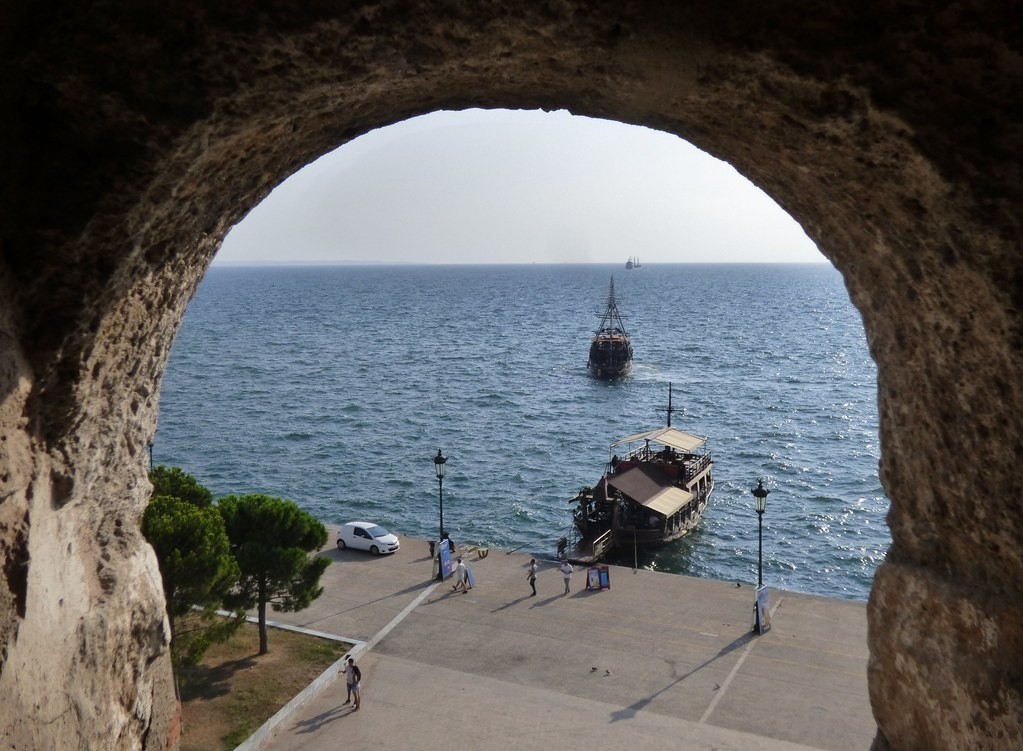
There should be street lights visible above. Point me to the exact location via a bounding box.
[750,479,772,634]
[431,449,450,580]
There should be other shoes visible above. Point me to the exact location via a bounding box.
[564,593,566,595]
[567,591,570,593]
[463,590,467,593]
[452,585,457,590]
[531,592,536,596]
[354,706,359,710]
[344,700,351,705]
[352,704,356,707]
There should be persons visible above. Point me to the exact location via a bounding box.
[452,559,467,593]
[560,560,573,594]
[527,559,537,596]
[612,446,684,529]
[338,659,362,711]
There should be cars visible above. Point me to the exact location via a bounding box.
[335,522,400,556]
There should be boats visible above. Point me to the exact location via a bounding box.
[625,256,642,270]
[587,273,634,380]
[568,382,715,548]
